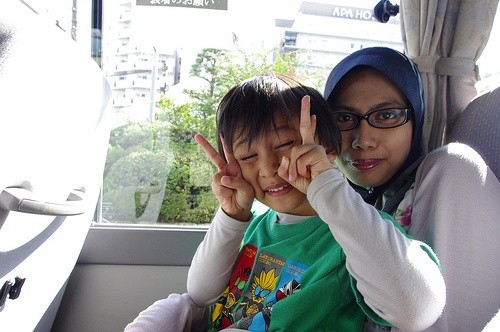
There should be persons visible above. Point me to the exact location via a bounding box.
[186,73,447,332]
[121,47,500,332]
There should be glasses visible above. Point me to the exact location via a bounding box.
[329,106,413,132]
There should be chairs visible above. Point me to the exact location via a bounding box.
[0,0,114,332]
[393,86,500,331]
